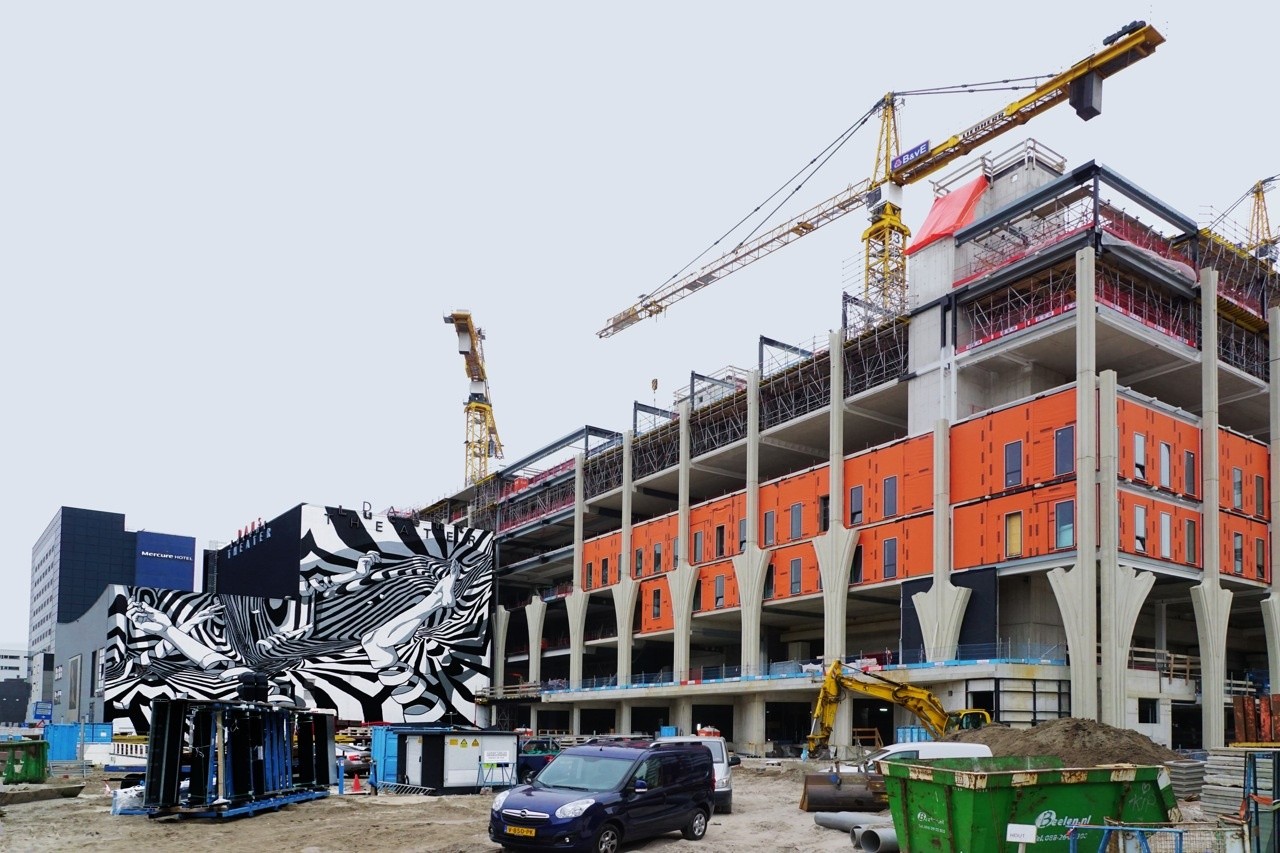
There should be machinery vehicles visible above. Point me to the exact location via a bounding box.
[805,660,993,764]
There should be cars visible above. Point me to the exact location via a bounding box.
[292,742,371,778]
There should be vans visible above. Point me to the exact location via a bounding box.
[658,736,742,813]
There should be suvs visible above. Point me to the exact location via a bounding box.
[487,740,717,853]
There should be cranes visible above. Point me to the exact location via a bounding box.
[596,18,1167,323]
[441,310,504,489]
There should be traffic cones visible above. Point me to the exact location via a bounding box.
[351,774,364,792]
